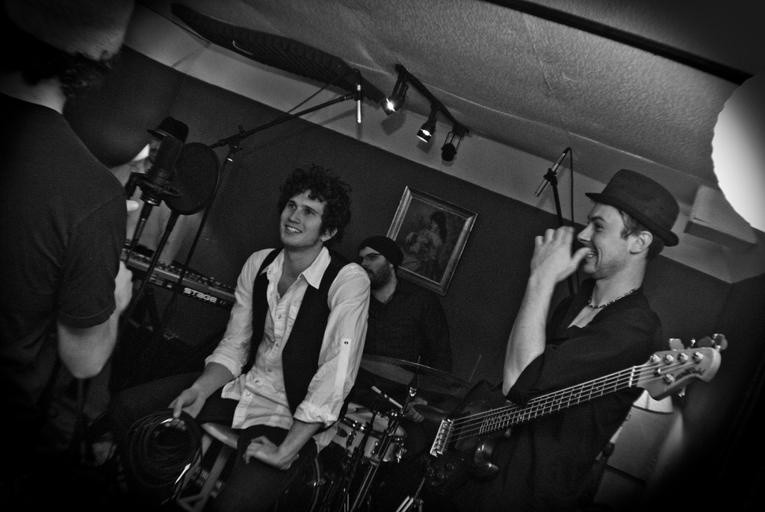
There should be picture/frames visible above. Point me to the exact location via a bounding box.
[385,184,479,295]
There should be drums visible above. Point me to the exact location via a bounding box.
[282,452,341,510]
[334,402,407,464]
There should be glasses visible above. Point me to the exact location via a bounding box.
[358,252,385,262]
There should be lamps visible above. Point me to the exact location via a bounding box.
[384,63,470,162]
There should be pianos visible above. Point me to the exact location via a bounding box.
[121,246,235,308]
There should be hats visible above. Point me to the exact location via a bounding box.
[357,235,402,264]
[4,0,136,65]
[147,116,189,146]
[584,168,680,247]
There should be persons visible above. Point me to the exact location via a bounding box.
[1,1,135,511]
[320,235,455,512]
[117,164,373,510]
[399,209,449,278]
[457,170,679,510]
[108,117,192,315]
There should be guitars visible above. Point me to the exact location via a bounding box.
[420,332,727,494]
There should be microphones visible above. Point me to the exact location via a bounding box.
[534,146,570,198]
[136,135,184,204]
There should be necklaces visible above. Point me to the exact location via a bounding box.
[585,288,636,310]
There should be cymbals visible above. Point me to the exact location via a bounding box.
[363,353,470,395]
[413,404,448,422]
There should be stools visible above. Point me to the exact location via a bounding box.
[160,421,240,512]
